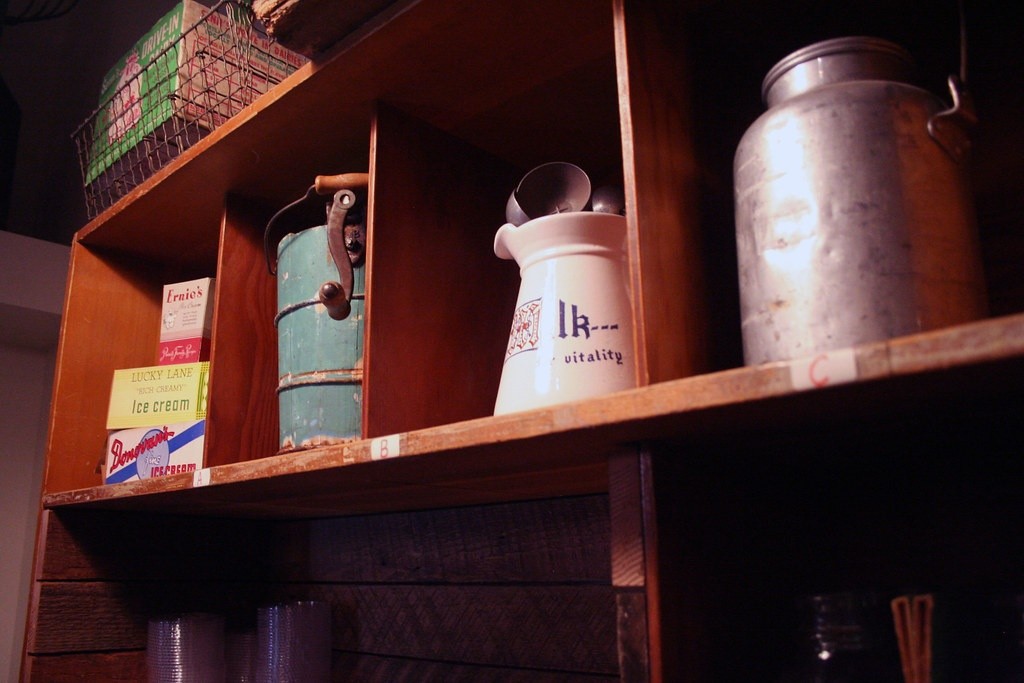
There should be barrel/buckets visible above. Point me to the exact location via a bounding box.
[729,36,987,369]
[263,173,372,452]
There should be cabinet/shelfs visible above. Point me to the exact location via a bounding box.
[20,0,1024,683]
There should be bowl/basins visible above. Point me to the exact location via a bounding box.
[147,597,338,683]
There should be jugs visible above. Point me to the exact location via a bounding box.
[488,210,640,418]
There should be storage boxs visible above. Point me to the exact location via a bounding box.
[65,2,983,485]
